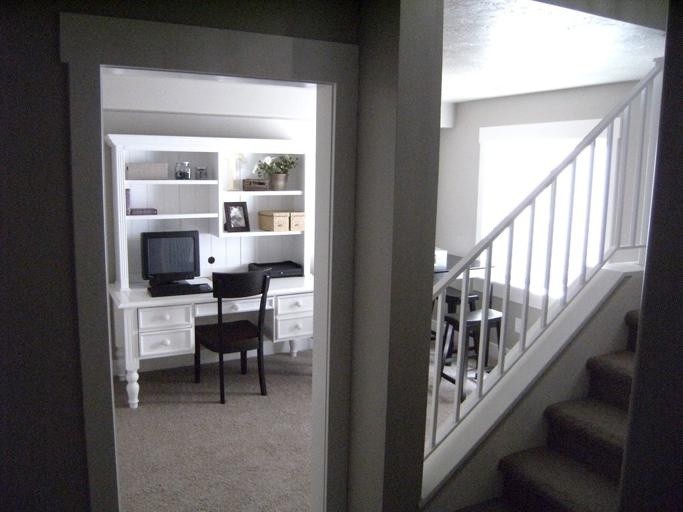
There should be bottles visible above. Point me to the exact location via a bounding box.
[174,161,209,180]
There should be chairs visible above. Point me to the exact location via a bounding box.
[194,267,273,404]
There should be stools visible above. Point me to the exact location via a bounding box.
[445,286,479,366]
[441,308,503,403]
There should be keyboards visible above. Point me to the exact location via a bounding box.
[148,284,212,297]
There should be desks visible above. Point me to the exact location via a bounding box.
[434,253,495,273]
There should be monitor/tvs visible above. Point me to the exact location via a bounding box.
[141,231,200,286]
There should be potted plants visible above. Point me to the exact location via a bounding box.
[258,155,299,189]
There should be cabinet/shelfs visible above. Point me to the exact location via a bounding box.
[105,133,315,410]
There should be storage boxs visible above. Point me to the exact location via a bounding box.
[289,211,305,231]
[258,210,289,232]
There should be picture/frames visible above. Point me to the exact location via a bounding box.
[224,202,251,233]
